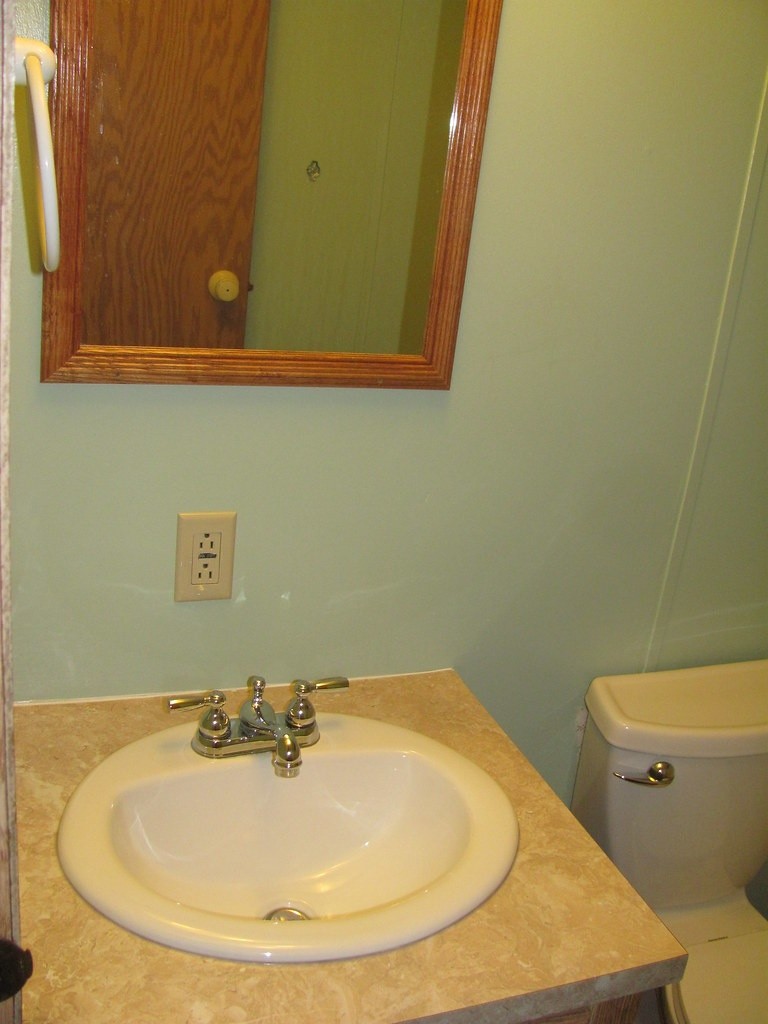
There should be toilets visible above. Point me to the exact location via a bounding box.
[569,659,768,1023]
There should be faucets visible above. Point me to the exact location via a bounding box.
[165,676,355,781]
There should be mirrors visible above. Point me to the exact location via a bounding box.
[40,0,505,391]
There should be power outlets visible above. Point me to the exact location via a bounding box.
[175,509,237,602]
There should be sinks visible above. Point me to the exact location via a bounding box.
[52,711,524,965]
